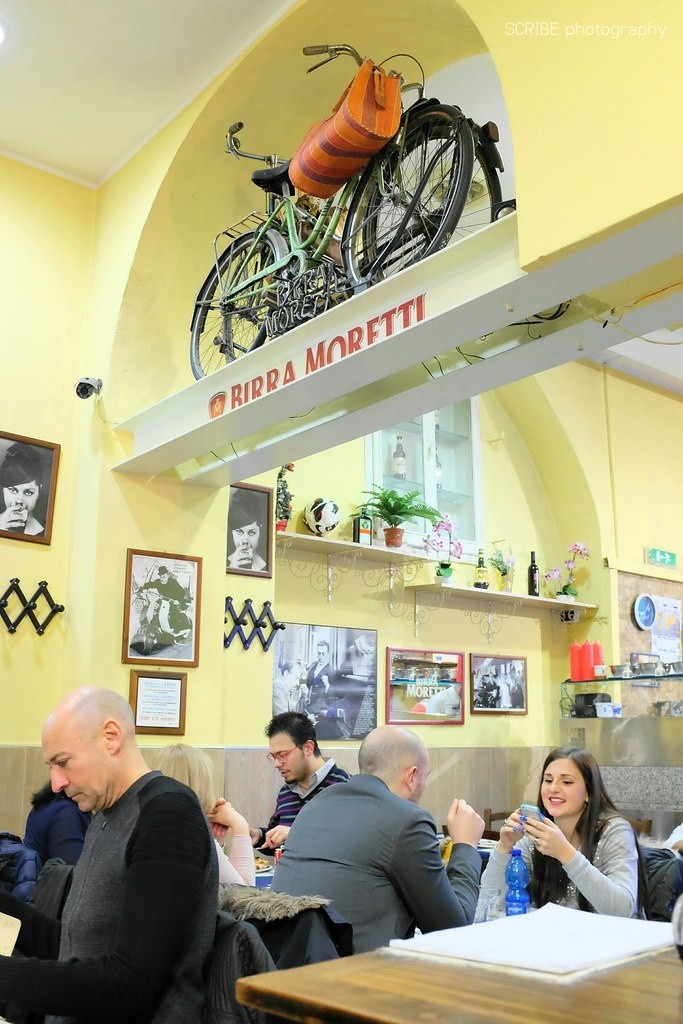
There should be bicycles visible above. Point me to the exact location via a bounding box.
[191,43,505,383]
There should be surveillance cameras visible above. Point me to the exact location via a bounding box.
[74,378,99,399]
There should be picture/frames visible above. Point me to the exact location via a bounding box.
[130,668,188,736]
[630,652,662,688]
[120,547,203,668]
[0,430,61,547]
[225,481,273,579]
[386,646,466,726]
[469,652,529,716]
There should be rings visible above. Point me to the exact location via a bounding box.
[534,837,538,843]
[502,819,506,826]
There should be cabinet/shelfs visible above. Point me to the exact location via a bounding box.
[405,584,600,649]
[364,395,485,568]
[276,531,436,609]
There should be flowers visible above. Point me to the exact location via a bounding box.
[543,540,592,598]
[486,550,516,579]
[420,511,469,561]
[276,462,297,519]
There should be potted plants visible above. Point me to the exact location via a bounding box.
[350,483,444,548]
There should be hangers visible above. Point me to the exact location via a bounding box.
[0,577,66,635]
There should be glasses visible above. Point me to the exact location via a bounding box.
[266,738,314,764]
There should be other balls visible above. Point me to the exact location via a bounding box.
[303,498,341,534]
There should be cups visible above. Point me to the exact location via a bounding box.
[671,661,683,674]
[610,665,625,677]
[638,663,657,675]
[594,665,607,680]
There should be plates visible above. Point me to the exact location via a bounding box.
[477,843,497,848]
[255,865,273,873]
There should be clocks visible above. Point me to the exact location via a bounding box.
[634,592,657,631]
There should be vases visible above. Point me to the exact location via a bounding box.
[276,520,287,532]
[434,576,456,586]
[556,594,577,603]
[493,567,515,594]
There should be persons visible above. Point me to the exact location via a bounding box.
[136,566,185,645]
[481,746,646,921]
[271,725,485,964]
[24,779,93,866]
[0,686,219,1024]
[411,672,523,715]
[666,821,683,855]
[158,744,255,888]
[274,636,375,739]
[0,443,45,539]
[228,490,267,573]
[248,712,351,857]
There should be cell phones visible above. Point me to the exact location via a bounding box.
[521,804,543,822]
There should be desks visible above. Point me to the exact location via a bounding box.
[234,901,683,1024]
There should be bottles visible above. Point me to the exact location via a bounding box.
[473,548,489,589]
[392,436,406,480]
[352,506,372,546]
[434,409,439,429]
[505,848,531,916]
[435,447,441,489]
[570,638,605,682]
[528,550,539,596]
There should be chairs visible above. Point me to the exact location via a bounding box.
[202,883,355,1024]
[442,808,653,843]
